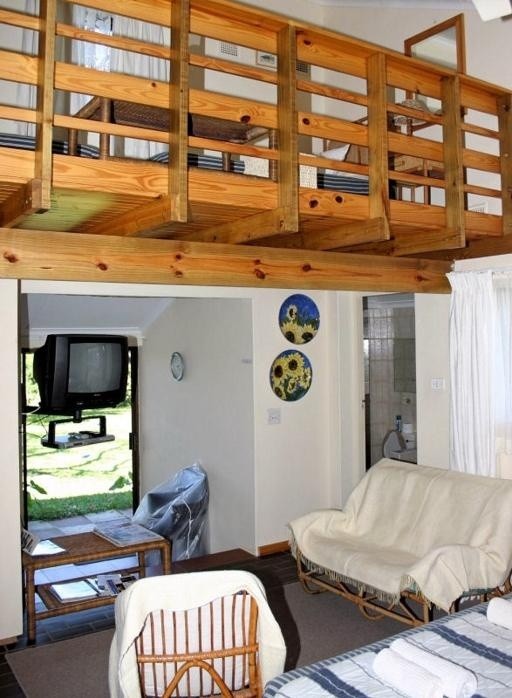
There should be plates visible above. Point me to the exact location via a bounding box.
[278,294,320,345]
[269,349,313,401]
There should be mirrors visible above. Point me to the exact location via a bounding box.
[393,338,416,394]
[403,14,468,132]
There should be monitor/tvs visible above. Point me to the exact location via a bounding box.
[33,334,128,411]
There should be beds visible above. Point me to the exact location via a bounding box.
[144,115,397,202]
[0,131,101,162]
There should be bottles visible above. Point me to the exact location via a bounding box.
[396,415,401,432]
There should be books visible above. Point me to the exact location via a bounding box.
[92,516,165,549]
[20,526,66,557]
[48,573,137,604]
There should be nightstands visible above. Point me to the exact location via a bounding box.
[393,153,446,205]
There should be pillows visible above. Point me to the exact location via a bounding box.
[320,144,351,162]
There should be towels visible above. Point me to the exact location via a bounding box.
[373,648,444,698]
[389,638,478,698]
[487,596,512,633]
[107,569,287,698]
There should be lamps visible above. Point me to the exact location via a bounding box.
[393,101,430,136]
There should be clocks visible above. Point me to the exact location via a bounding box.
[171,352,184,382]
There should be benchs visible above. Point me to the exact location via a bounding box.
[263,593,512,698]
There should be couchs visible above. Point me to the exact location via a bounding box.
[286,458,512,628]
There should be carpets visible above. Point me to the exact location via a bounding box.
[4,574,479,698]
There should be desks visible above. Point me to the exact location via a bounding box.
[23,532,172,643]
[67,95,279,184]
